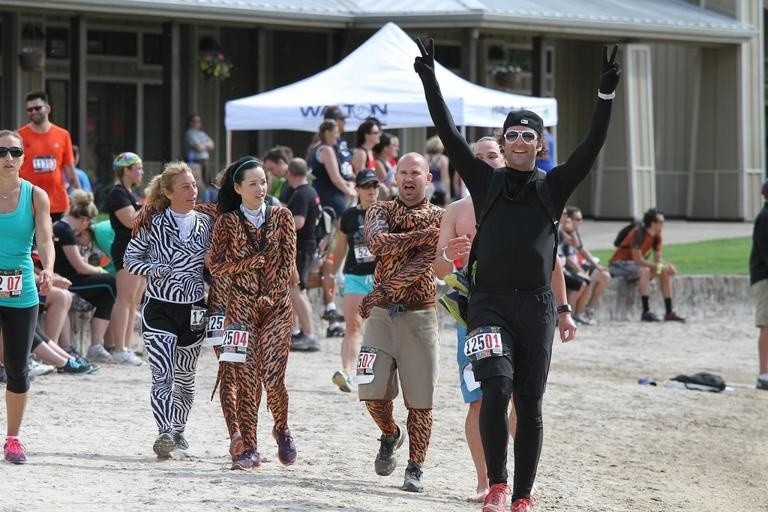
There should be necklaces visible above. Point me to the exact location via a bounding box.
[0,182,20,201]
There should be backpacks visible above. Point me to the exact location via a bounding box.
[614,222,644,249]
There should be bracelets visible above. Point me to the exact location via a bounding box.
[374,280,388,298]
[595,88,618,102]
[555,300,573,316]
[441,243,454,266]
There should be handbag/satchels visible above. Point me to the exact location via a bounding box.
[670,371,726,393]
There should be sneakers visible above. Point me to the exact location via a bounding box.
[152,427,175,460]
[231,450,262,473]
[402,460,424,493]
[374,423,407,476]
[0,345,143,381]
[482,483,512,512]
[511,498,533,512]
[229,433,243,462]
[757,378,767,389]
[4,439,27,464]
[290,310,354,350]
[271,425,297,467]
[572,310,685,327]
[174,433,189,451]
[332,369,354,395]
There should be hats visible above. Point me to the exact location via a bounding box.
[113,152,142,170]
[356,169,378,185]
[502,110,544,136]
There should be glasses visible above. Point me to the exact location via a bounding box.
[26,106,41,112]
[359,183,379,189]
[0,146,23,158]
[504,130,538,145]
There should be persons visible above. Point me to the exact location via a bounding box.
[1,90,150,383]
[0,126,56,465]
[191,168,302,460]
[415,25,622,510]
[354,150,444,494]
[433,130,517,504]
[182,106,400,392]
[121,158,214,458]
[423,129,687,328]
[746,180,767,391]
[204,156,299,470]
